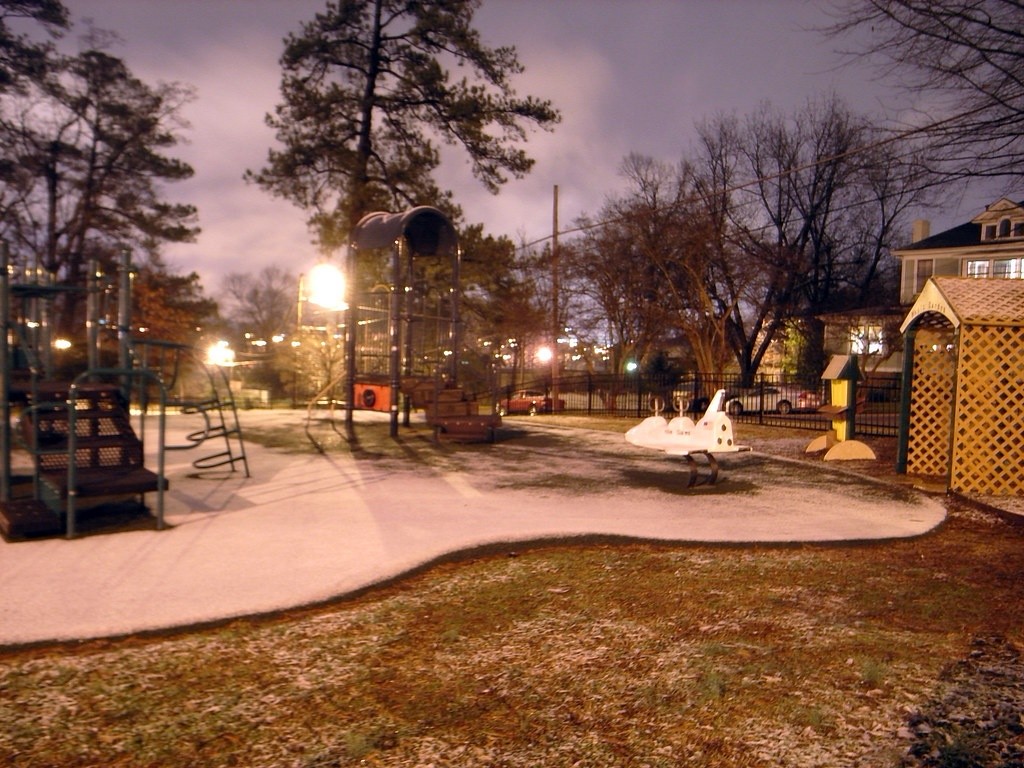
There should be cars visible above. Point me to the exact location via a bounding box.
[681,387,743,412]
[495,390,566,417]
[725,386,821,416]
